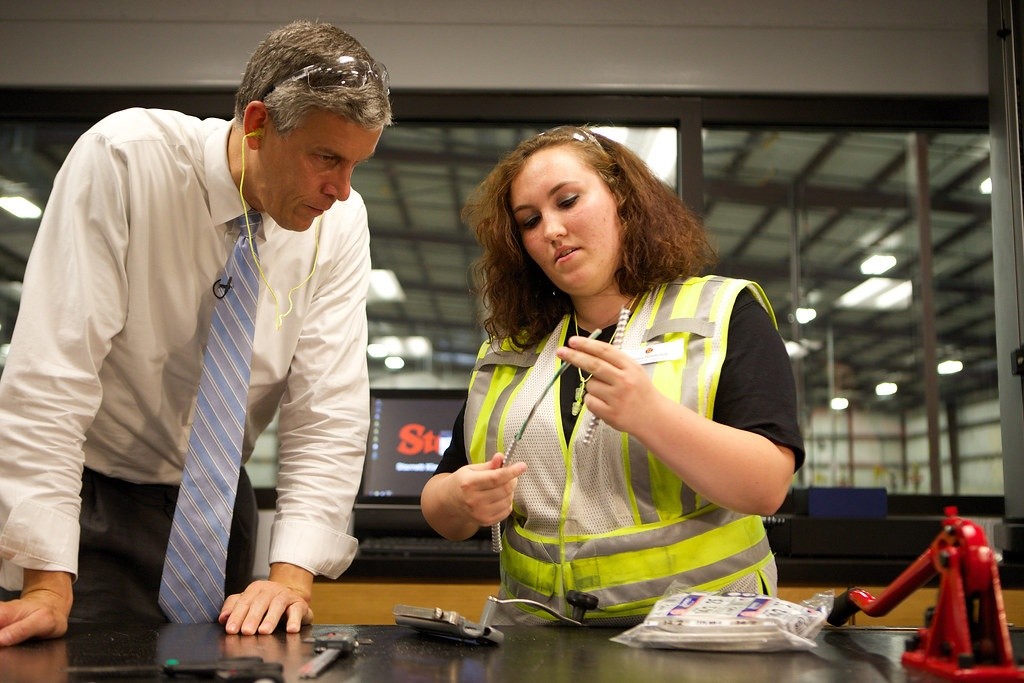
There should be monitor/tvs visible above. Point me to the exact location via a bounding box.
[354,387,469,510]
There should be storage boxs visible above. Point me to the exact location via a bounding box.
[761,485,945,558]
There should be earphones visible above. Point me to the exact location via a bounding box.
[248,128,265,140]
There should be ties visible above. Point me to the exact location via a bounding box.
[159,211,261,627]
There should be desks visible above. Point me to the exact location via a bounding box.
[1,620,1024,683]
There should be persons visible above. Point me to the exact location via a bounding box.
[0,19,393,646]
[423,124,807,626]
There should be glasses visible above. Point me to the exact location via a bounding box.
[533,125,611,157]
[260,57,391,98]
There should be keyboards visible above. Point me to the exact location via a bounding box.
[356,537,500,555]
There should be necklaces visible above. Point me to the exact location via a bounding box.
[572,292,638,416]
